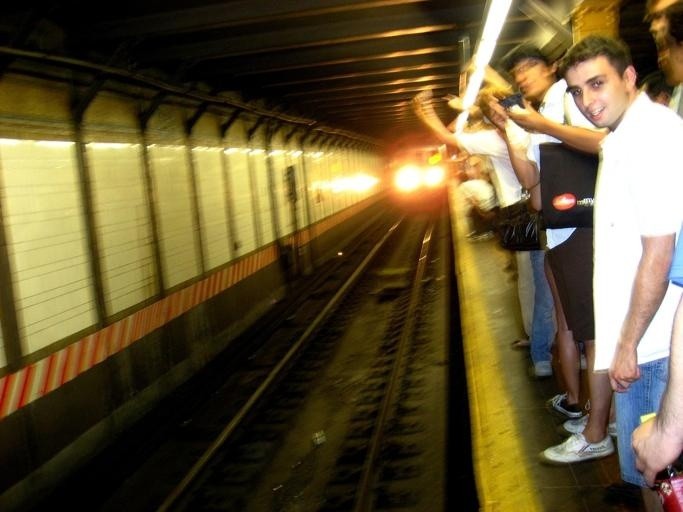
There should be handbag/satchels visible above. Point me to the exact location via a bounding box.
[491,189,547,251]
[540,141,600,231]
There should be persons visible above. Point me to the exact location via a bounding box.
[409,0,683,511]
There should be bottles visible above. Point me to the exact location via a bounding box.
[491,89,524,113]
[637,413,683,511]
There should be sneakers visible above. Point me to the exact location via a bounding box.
[533,359,554,377]
[563,413,620,435]
[553,393,583,419]
[544,434,616,463]
